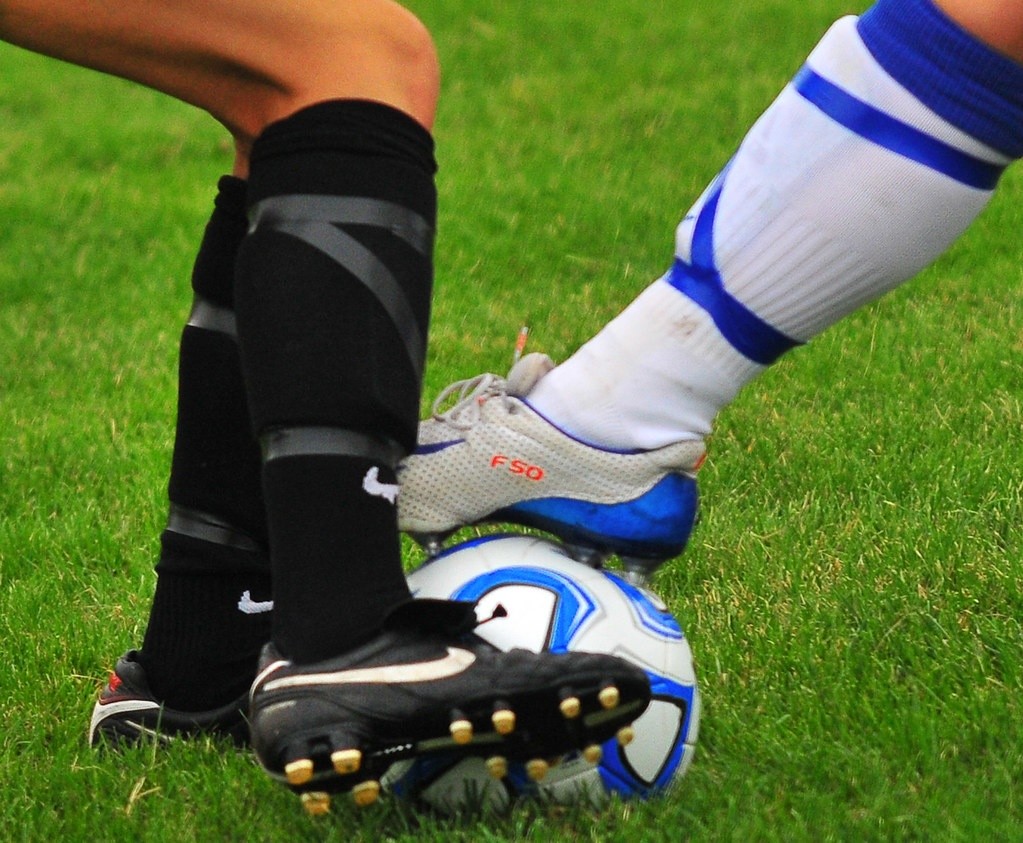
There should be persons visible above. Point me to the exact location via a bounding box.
[1,1,651,817]
[396,0,1023,578]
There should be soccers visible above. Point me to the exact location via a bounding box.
[380,533,701,810]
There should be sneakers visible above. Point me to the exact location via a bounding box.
[393,328,697,587]
[248,599,651,817]
[89,649,250,754]
